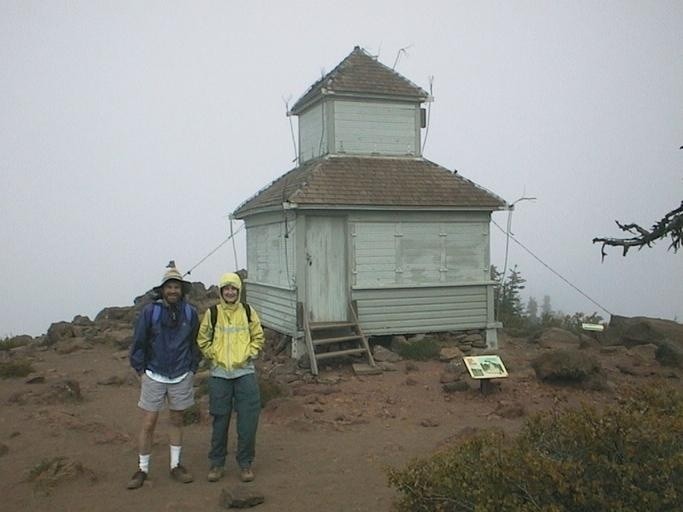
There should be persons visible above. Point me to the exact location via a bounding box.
[197,273,266,482]
[126,269,203,488]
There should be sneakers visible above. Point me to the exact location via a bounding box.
[168,464,193,482]
[127,470,147,489]
[208,468,224,481]
[240,469,254,481]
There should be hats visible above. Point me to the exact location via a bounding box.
[153,268,192,296]
